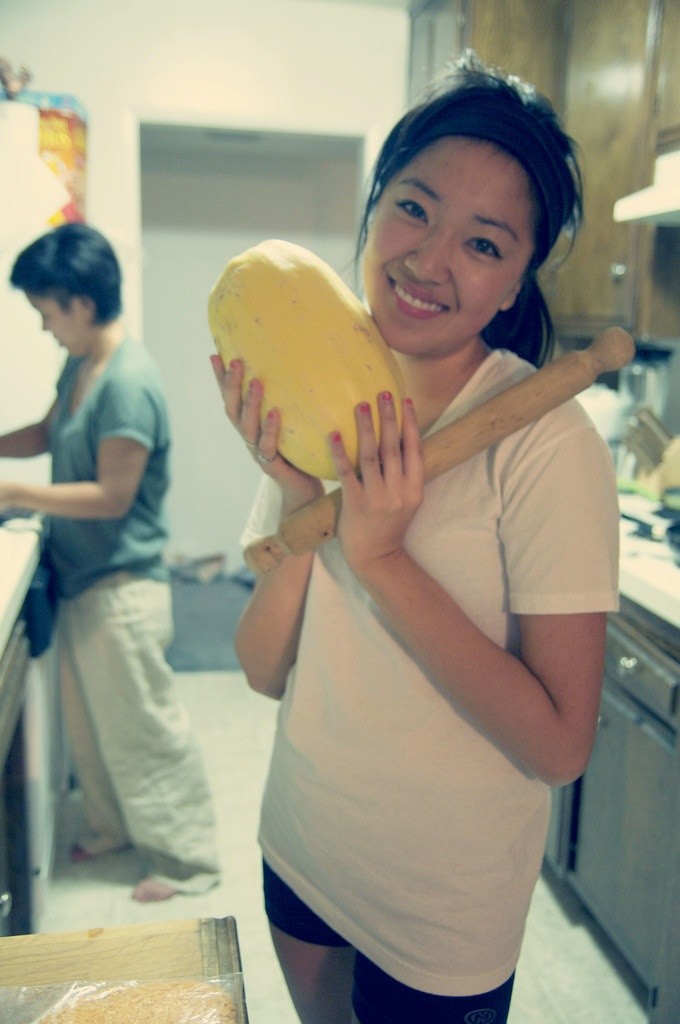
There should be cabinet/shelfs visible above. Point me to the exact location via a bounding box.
[540,540,680,1024]
[460,0,680,353]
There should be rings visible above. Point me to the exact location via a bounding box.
[245,440,256,448]
[257,453,275,464]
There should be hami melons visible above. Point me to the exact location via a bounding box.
[207,238,408,481]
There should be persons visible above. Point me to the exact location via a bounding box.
[208,50,619,1024]
[0,222,224,902]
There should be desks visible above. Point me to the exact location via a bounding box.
[0,916,251,1024]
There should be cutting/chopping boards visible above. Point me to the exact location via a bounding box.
[0,915,250,1024]
